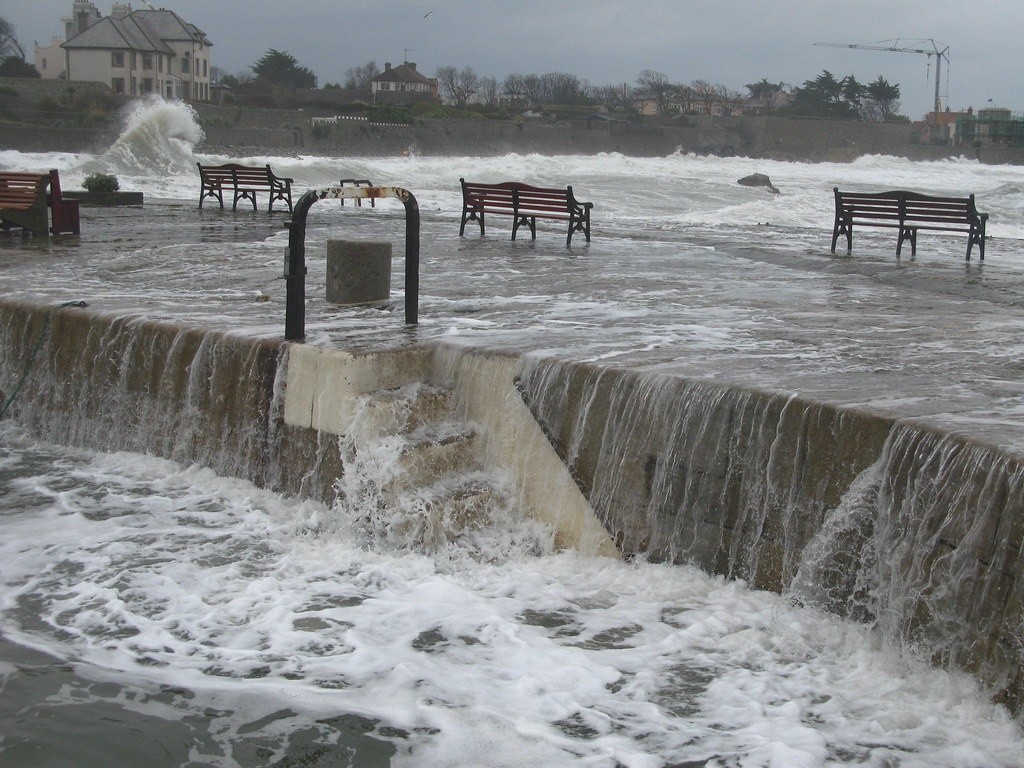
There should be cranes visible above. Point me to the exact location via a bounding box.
[812,36,951,129]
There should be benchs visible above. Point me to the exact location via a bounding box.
[0,168,82,239]
[830,187,989,261]
[196,162,294,214]
[459,178,594,247]
